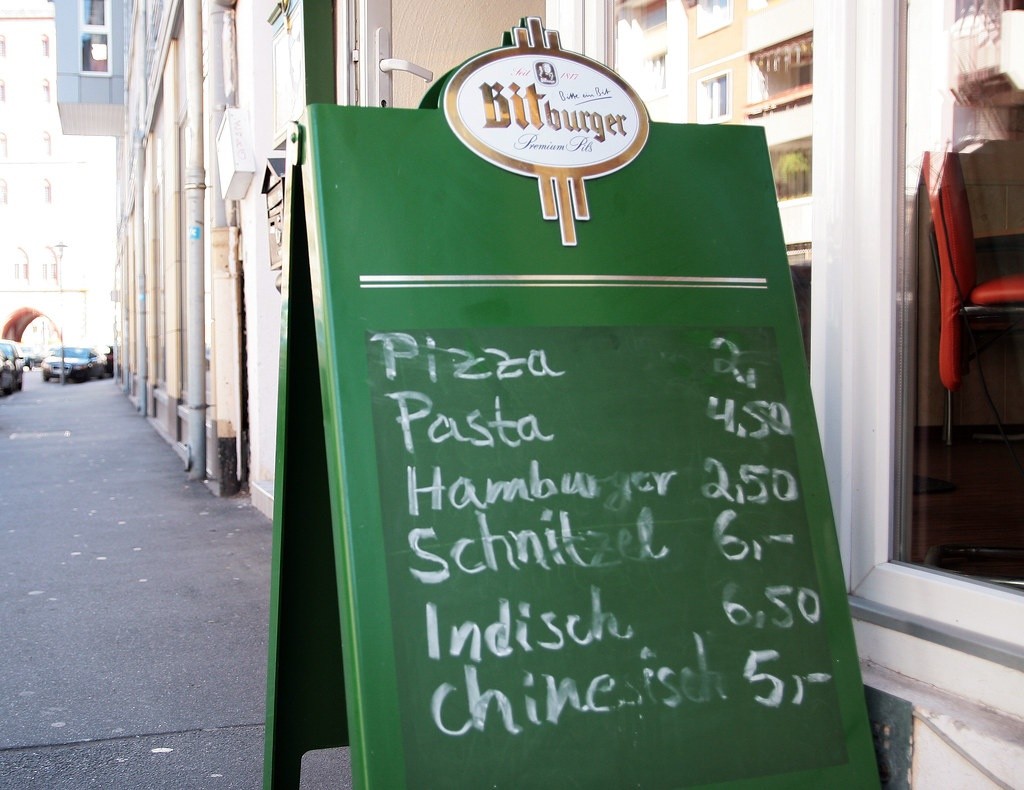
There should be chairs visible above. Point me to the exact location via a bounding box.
[927,135,1024,446]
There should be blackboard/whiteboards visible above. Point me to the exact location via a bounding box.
[264,103,879,790]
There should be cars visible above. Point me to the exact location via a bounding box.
[40,344,109,383]
[1,338,44,398]
